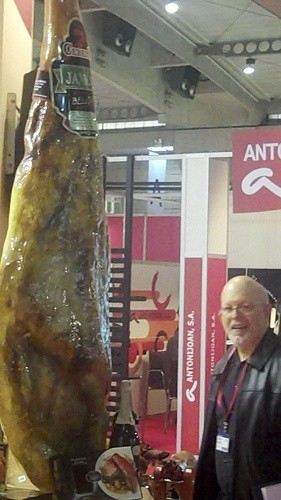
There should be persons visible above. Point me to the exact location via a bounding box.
[191,275,281,500]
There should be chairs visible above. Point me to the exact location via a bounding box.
[141,350,178,434]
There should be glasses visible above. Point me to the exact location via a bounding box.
[220,303,269,313]
